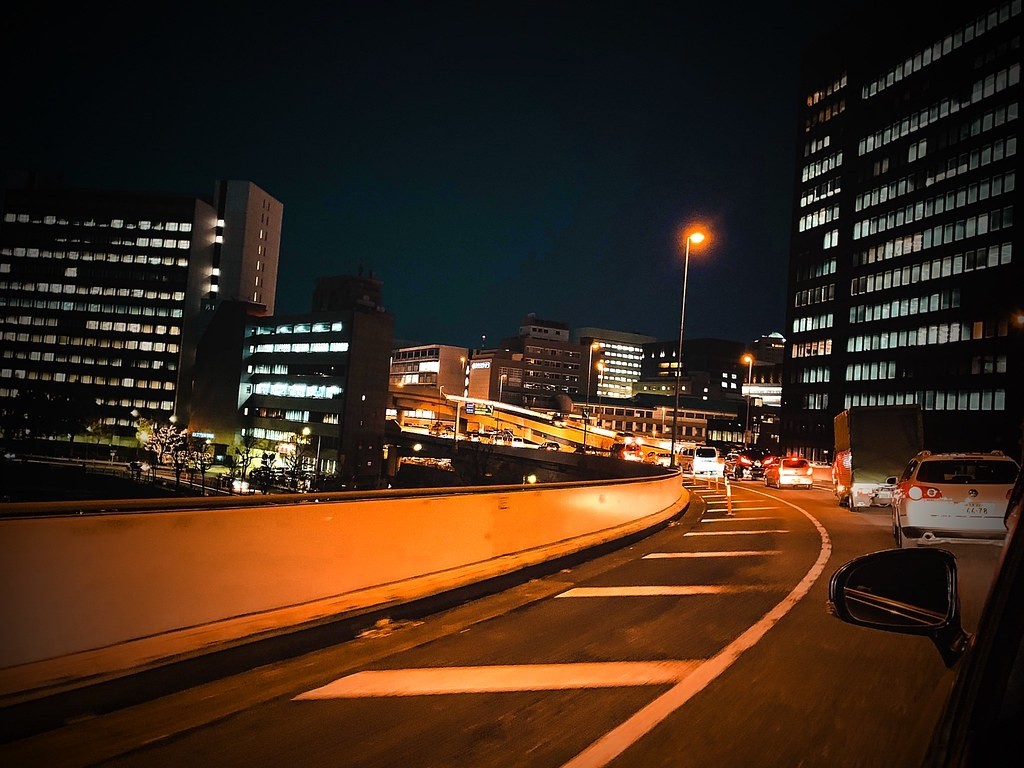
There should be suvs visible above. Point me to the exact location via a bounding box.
[723,448,771,480]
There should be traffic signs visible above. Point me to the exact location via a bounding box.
[465,400,496,417]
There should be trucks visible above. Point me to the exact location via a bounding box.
[833,403,928,513]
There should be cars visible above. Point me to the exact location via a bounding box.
[763,457,814,490]
[321,473,381,493]
[538,443,561,453]
[645,451,672,468]
[886,450,1021,549]
[431,424,447,435]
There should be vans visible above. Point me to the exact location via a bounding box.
[674,445,719,475]
[608,442,644,461]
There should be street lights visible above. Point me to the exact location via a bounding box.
[596,360,604,425]
[742,353,754,449]
[499,373,509,405]
[303,427,323,487]
[580,341,601,455]
[436,385,446,436]
[669,228,709,470]
